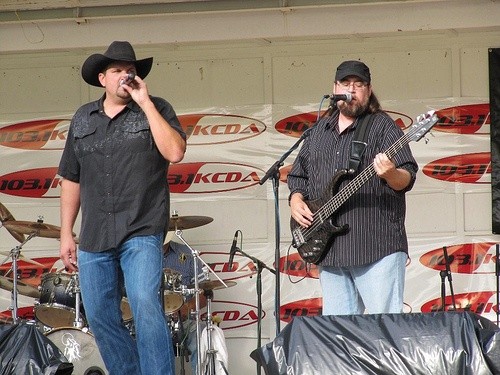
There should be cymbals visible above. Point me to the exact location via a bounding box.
[0,276,43,298]
[0,202,26,244]
[167,216,214,232]
[2,220,76,238]
[0,251,46,267]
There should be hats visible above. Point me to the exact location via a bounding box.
[81,40,153,87]
[335,60,370,83]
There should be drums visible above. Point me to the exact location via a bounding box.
[33,273,90,330]
[161,268,187,316]
[120,284,134,323]
[42,327,111,375]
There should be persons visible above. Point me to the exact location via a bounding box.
[288,61,418,316]
[57,41,186,374]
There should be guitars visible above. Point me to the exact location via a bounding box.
[289,108,441,263]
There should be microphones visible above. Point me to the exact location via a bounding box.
[324,93,352,103]
[120,73,135,87]
[228,232,239,271]
